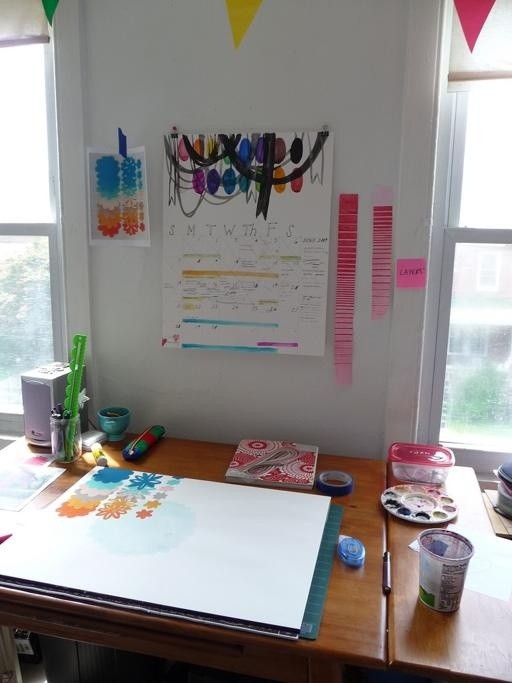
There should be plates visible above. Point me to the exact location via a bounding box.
[380,483,459,524]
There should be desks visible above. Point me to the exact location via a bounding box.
[1,434,511,683]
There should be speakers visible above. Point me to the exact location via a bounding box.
[21,361,88,448]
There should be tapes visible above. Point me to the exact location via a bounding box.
[316,469,354,496]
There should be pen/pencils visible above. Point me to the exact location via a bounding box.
[383,550,392,594]
[50,403,71,456]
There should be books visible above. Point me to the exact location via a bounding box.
[224,439,319,490]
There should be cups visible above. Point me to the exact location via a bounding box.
[97,406,130,441]
[416,528,474,612]
